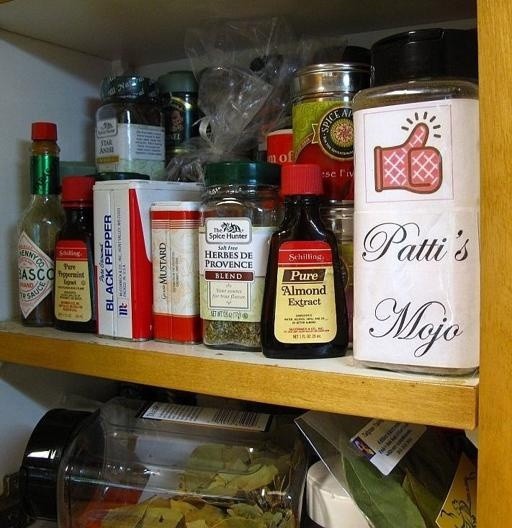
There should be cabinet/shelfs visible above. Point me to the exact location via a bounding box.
[0,0,512,528]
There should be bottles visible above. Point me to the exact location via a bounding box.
[21,392,307,527]
[199,27,479,378]
[95,68,200,180]
[12,118,95,333]
